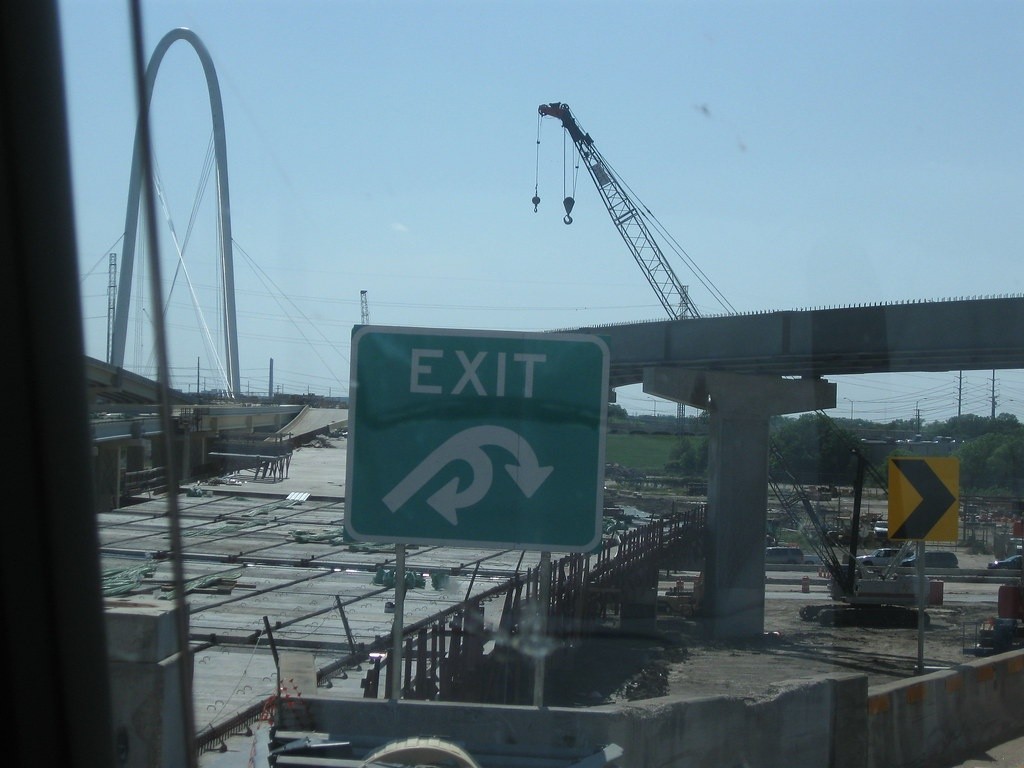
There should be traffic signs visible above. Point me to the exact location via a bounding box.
[343,324,612,556]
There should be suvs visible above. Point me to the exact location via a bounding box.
[853,548,913,566]
[902,549,959,569]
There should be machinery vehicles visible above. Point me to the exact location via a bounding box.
[530,99,934,630]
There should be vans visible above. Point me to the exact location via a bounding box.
[765,547,803,564]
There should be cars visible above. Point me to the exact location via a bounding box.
[874,520,888,537]
[986,555,1024,570]
